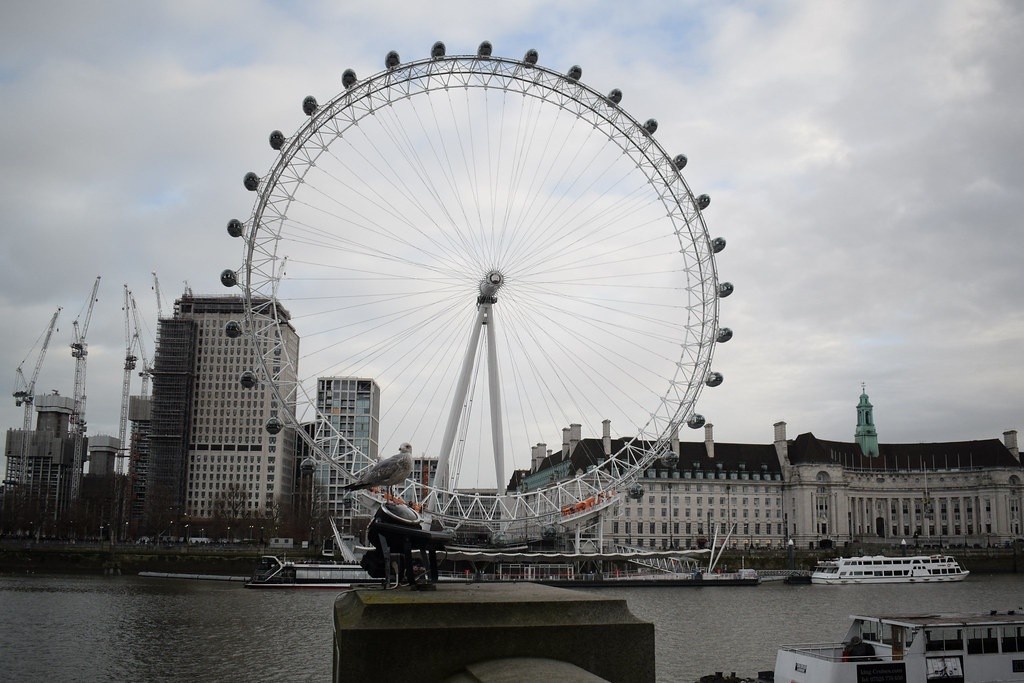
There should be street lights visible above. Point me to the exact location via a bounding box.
[667,483,672,546]
[726,485,731,550]
[781,486,787,549]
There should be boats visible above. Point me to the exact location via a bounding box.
[810,551,972,585]
[242,546,761,587]
[772,612,1024,683]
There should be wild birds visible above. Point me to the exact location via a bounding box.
[340,443,414,506]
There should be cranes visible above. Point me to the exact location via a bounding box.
[12,270,163,524]
